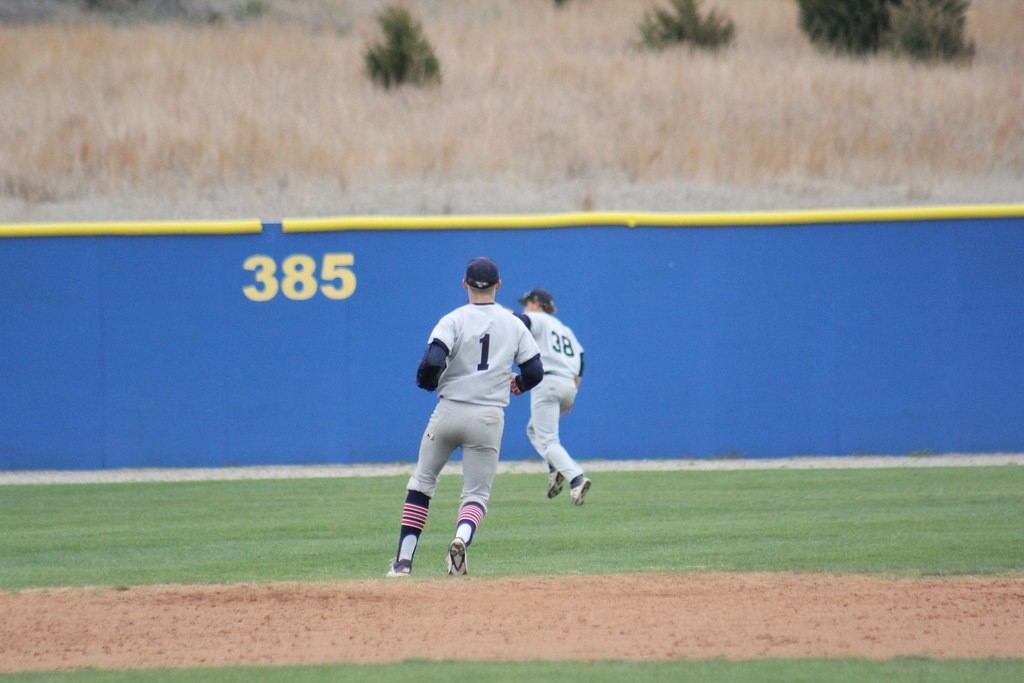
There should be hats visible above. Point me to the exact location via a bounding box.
[465,257,499,290]
[518,288,553,305]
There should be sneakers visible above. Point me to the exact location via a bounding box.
[547,470,565,499]
[446,537,467,576]
[570,477,592,506]
[386,558,412,578]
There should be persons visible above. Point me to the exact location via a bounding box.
[503,289,590,505]
[387,258,543,578]
